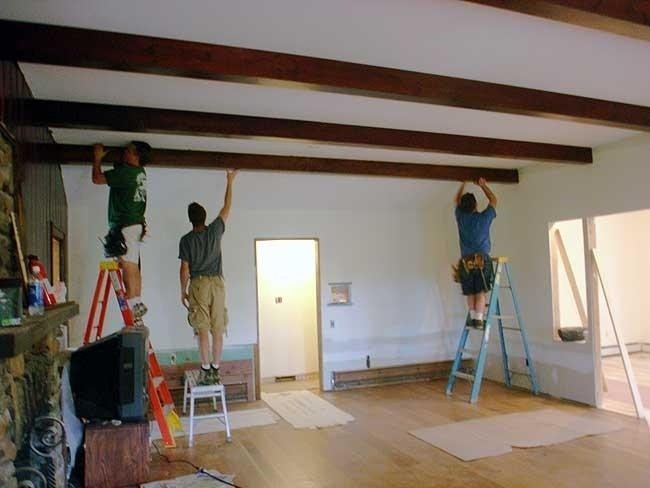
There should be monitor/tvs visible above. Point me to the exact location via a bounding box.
[69,325,148,423]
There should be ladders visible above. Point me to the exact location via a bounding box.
[83,261,183,448]
[446,256,539,404]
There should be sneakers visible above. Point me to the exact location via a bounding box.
[466,318,485,329]
[132,302,148,318]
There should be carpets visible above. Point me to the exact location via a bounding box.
[262,385,357,432]
[139,468,235,488]
[145,406,277,452]
[406,409,625,464]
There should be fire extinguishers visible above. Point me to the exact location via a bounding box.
[25,251,56,307]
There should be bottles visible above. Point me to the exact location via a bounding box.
[27,265,45,316]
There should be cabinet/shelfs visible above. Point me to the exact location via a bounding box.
[82,420,151,487]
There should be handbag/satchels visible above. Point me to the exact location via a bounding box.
[104,228,128,257]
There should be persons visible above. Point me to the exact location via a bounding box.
[452,177,497,329]
[178,168,237,385]
[92,141,153,318]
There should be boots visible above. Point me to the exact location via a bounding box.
[198,362,220,385]
